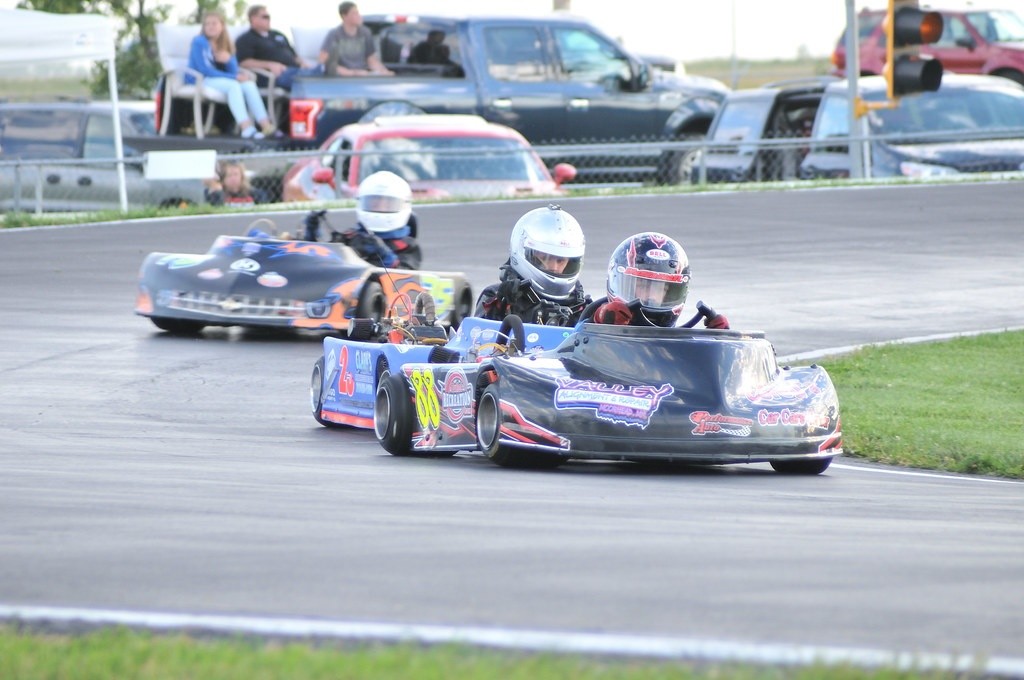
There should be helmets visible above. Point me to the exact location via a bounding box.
[509,206,586,299]
[608,233,691,317]
[356,171,412,235]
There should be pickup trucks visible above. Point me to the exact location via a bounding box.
[155,13,730,189]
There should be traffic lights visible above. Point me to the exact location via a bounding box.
[881,0,944,97]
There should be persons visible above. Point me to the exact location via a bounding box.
[184,1,393,140]
[199,160,270,208]
[473,203,730,330]
[301,170,424,273]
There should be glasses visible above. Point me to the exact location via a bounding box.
[262,15,270,20]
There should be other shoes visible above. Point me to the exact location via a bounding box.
[241,126,287,141]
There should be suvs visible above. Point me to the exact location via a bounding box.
[0,94,262,216]
[690,77,840,185]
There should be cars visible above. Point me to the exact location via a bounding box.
[281,117,576,204]
[829,8,1024,88]
[798,74,1024,180]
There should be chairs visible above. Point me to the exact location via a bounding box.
[158,65,288,141]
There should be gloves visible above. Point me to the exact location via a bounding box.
[496,279,524,306]
[352,223,399,267]
[595,301,632,326]
[704,314,728,329]
